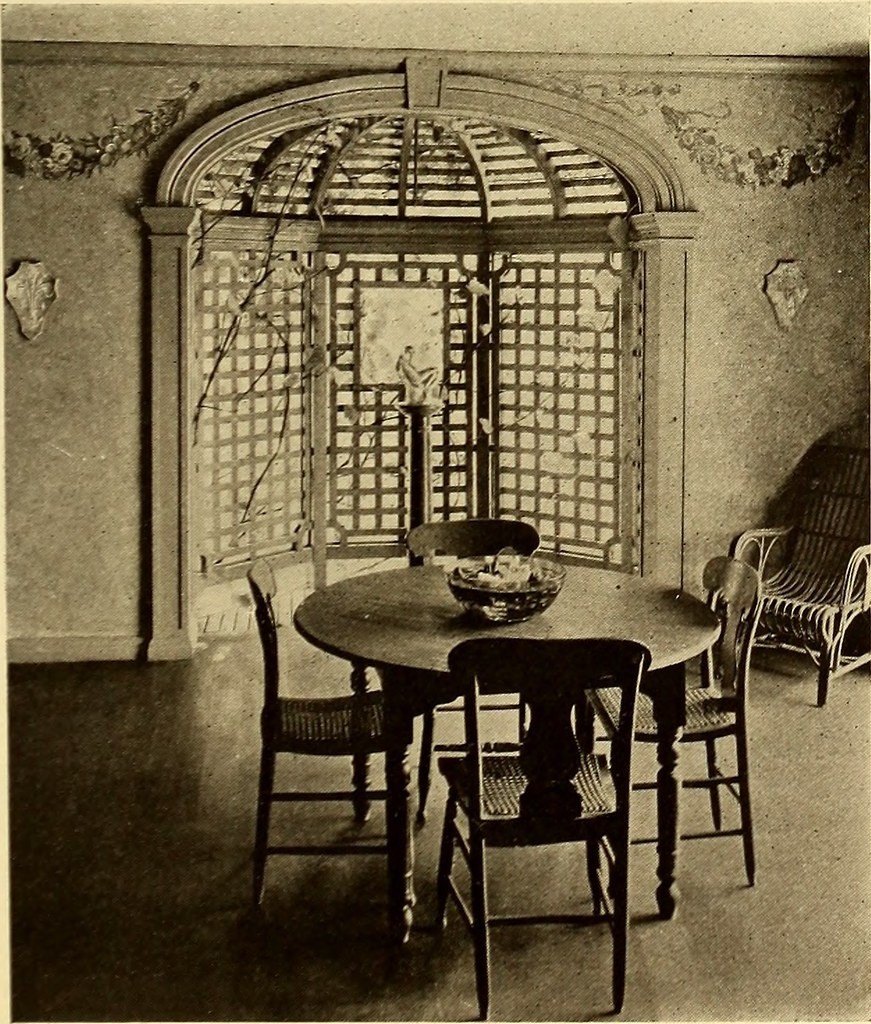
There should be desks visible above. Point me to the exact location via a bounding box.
[291,559,721,946]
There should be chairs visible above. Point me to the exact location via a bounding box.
[574,556,768,918]
[403,516,543,829]
[246,559,421,945]
[427,635,654,1021]
[707,408,871,708]
